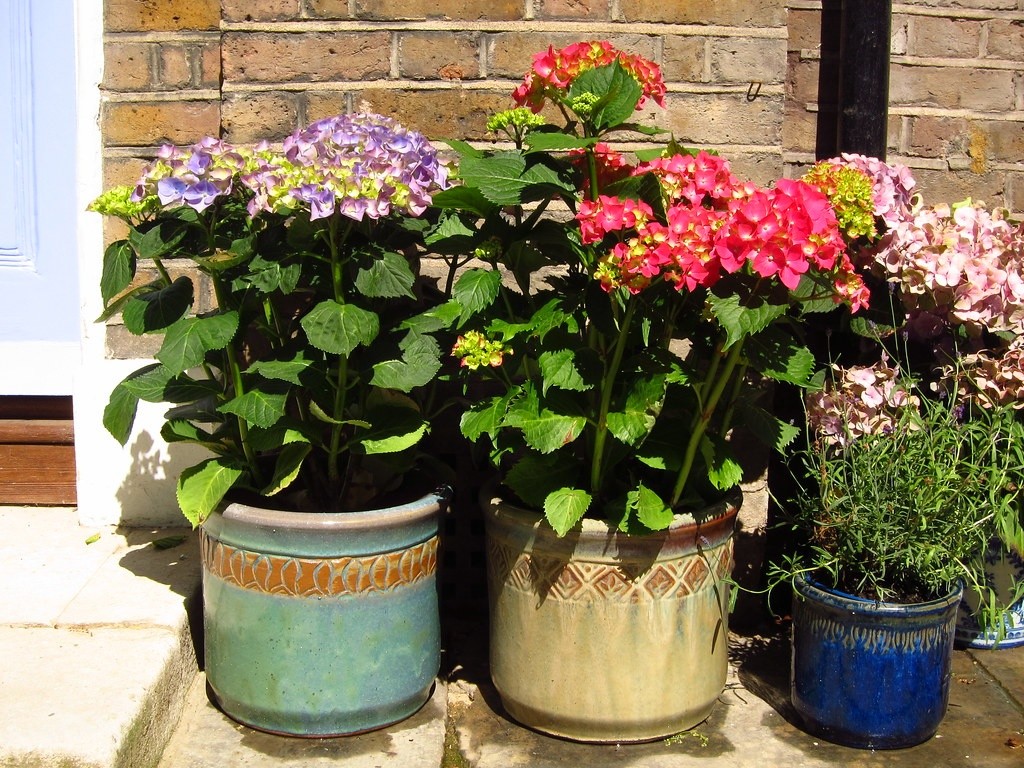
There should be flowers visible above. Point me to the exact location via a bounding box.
[84,101,455,532]
[708,313,1024,647]
[426,39,868,540]
[797,152,1024,455]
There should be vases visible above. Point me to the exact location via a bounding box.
[945,541,1024,636]
[200,479,440,739]
[480,484,744,746]
[790,562,963,751]
